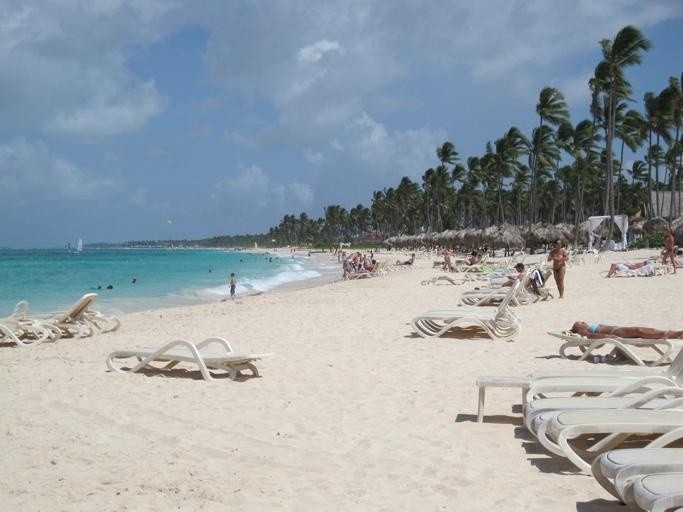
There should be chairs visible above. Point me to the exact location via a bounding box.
[0,292,118,350]
[547,329,674,368]
[102,336,271,382]
[474,347,682,511]
[601,252,681,278]
[344,253,416,281]
[410,250,604,343]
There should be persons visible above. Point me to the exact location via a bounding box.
[434,244,489,274]
[548,239,569,299]
[572,319,683,339]
[663,228,677,274]
[393,253,415,265]
[608,260,647,277]
[502,262,525,287]
[336,250,379,281]
[228,273,237,297]
